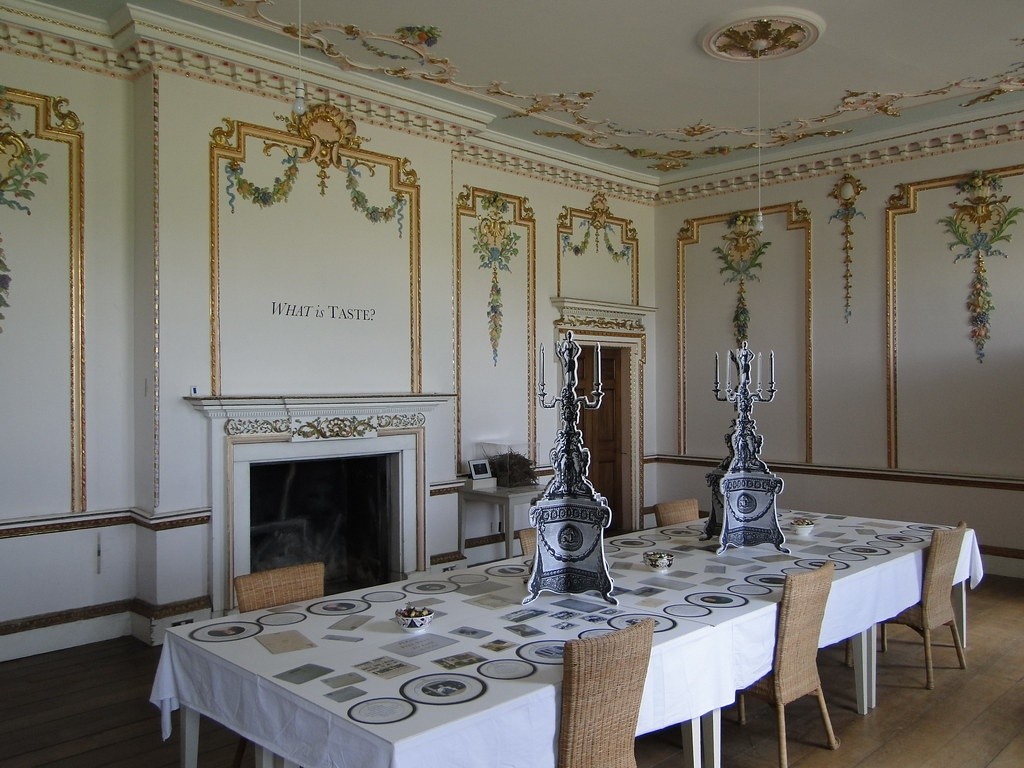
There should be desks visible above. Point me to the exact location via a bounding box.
[150,508,982,768]
[456,487,549,560]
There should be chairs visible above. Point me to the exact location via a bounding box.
[558,618,655,768]
[653,497,700,526]
[229,561,326,768]
[738,562,841,768]
[846,520,967,691]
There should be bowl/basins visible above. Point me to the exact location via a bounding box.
[642,551,675,572]
[790,521,815,536]
[395,607,436,636]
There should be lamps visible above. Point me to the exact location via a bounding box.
[696,6,827,235]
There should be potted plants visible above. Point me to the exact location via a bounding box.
[481,446,541,489]
